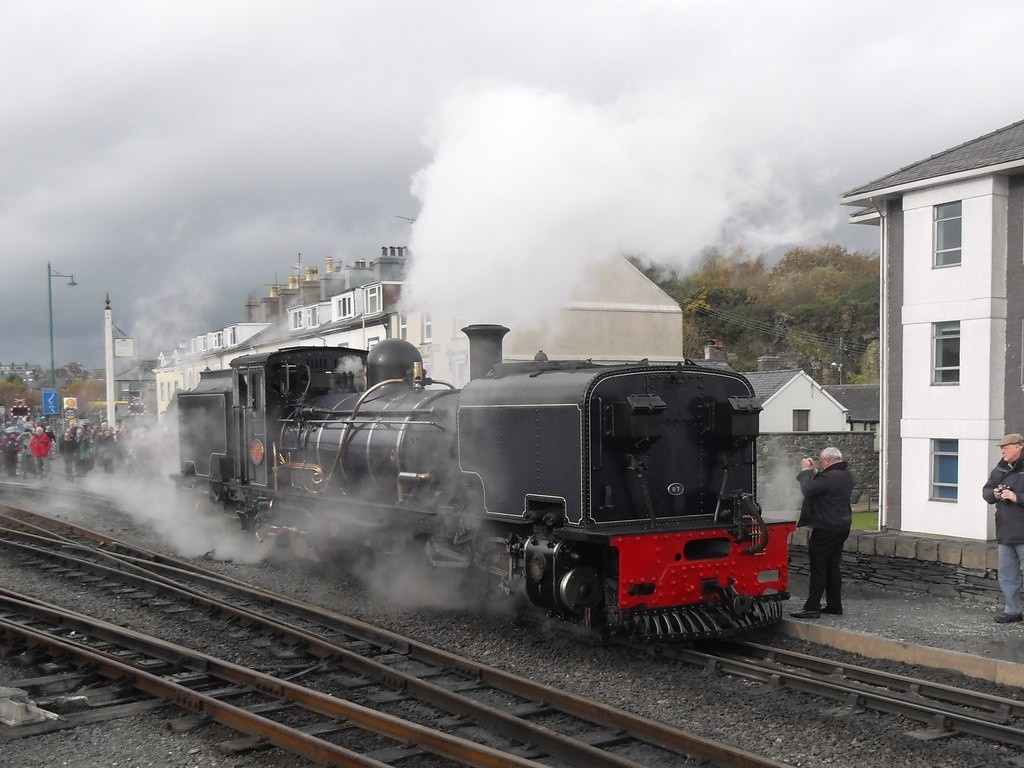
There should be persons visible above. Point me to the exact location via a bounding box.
[0,414,176,484]
[981,433,1024,624]
[789,446,855,621]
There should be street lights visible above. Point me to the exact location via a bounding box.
[47,261,79,464]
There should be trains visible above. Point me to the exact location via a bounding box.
[170,320,799,652]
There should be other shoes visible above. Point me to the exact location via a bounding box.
[821,603,843,615]
[994,612,1022,623]
[789,609,820,618]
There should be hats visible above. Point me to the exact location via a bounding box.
[996,433,1023,447]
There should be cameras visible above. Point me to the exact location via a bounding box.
[998,485,1013,504]
